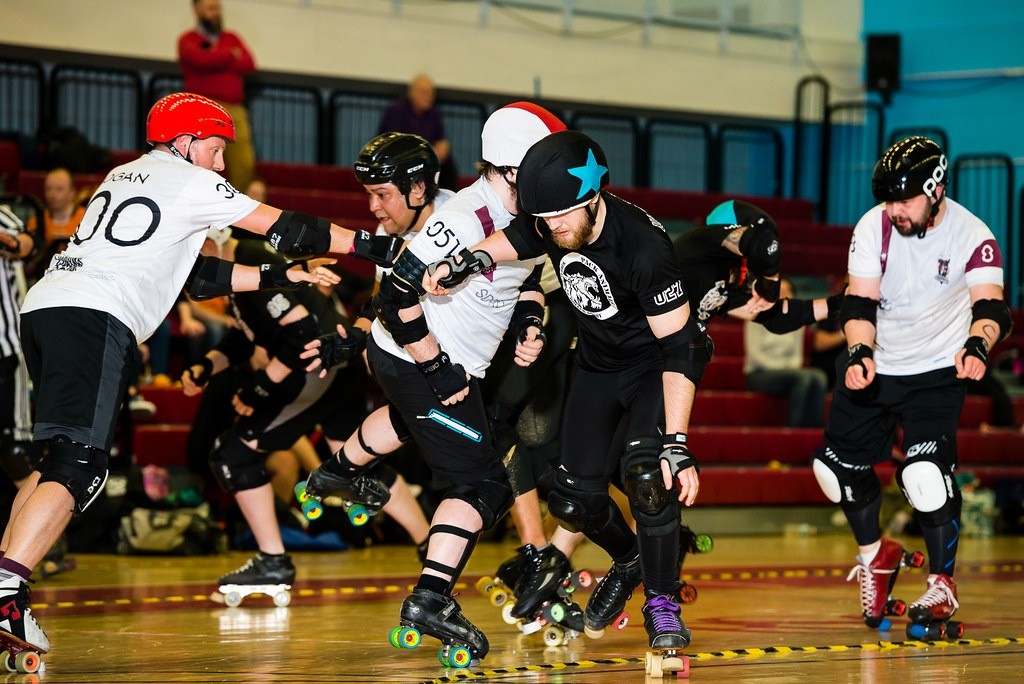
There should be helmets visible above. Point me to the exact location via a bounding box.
[705,199,773,227]
[481,101,567,167]
[146,92,236,146]
[518,131,610,217]
[353,132,440,201]
[871,135,948,201]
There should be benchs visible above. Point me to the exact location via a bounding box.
[0,143,1023,504]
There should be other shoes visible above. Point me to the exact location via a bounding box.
[41,553,77,576]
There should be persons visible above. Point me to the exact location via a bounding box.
[177,0,257,194]
[377,76,458,191]
[749,276,1010,433]
[0,93,410,679]
[808,133,1014,641]
[0,102,846,678]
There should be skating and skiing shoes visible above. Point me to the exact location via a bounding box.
[905,574,963,641]
[674,523,713,605]
[474,546,546,634]
[641,595,691,678]
[217,552,296,607]
[500,544,586,647]
[0,673,40,684]
[846,537,925,630]
[584,550,642,639]
[389,587,489,668]
[0,576,50,673]
[294,468,390,525]
